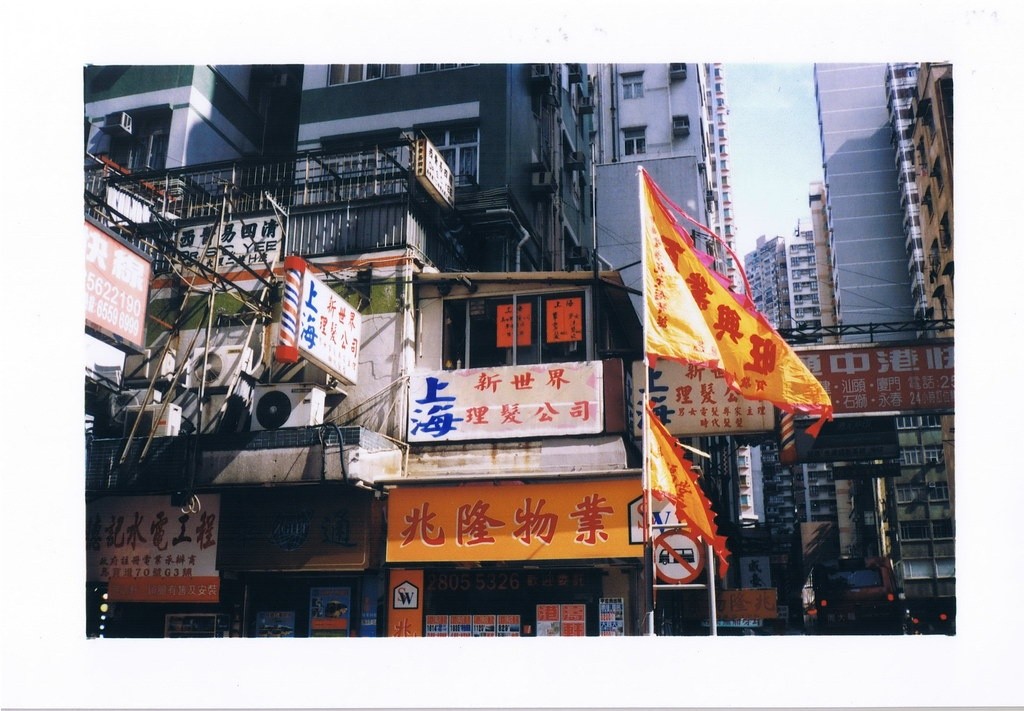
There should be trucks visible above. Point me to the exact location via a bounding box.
[812,556,903,627]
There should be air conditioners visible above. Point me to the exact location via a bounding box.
[928,482,936,487]
[190,345,254,389]
[573,247,589,261]
[250,383,325,431]
[105,111,131,134]
[124,403,181,437]
[674,121,684,128]
[580,97,594,115]
[572,152,586,165]
[931,457,937,462]
[531,64,552,84]
[108,389,162,422]
